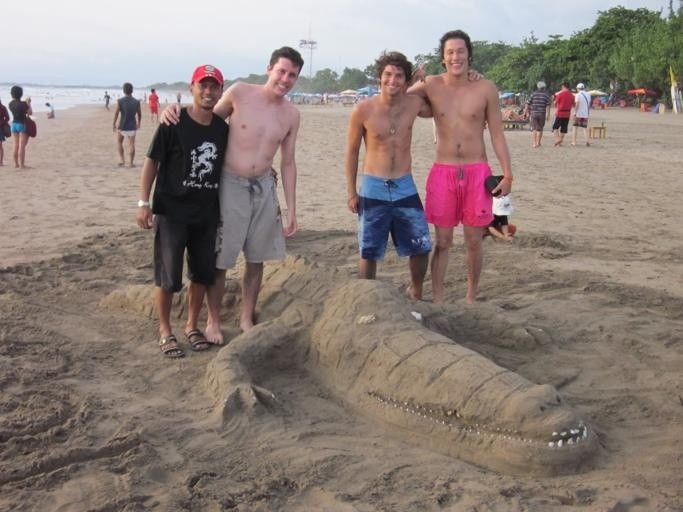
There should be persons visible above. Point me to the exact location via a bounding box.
[483,177,516,243]
[159,43,305,345]
[344,48,485,300]
[405,29,514,304]
[136,62,230,358]
[522,80,552,148]
[570,82,592,146]
[551,82,575,148]
[0,82,182,168]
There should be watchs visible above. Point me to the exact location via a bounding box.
[135,199,151,208]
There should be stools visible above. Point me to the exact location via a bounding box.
[589,126,606,139]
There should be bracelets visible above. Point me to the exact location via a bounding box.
[504,175,513,181]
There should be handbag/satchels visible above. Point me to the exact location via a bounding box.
[24,115,37,138]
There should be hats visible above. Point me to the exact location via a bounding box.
[192,63,227,86]
[536,80,547,89]
[575,82,586,89]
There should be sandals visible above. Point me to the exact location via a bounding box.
[158,335,184,359]
[184,328,210,353]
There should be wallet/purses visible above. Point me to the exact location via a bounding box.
[486,175,506,198]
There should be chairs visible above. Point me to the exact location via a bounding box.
[502,114,530,131]
